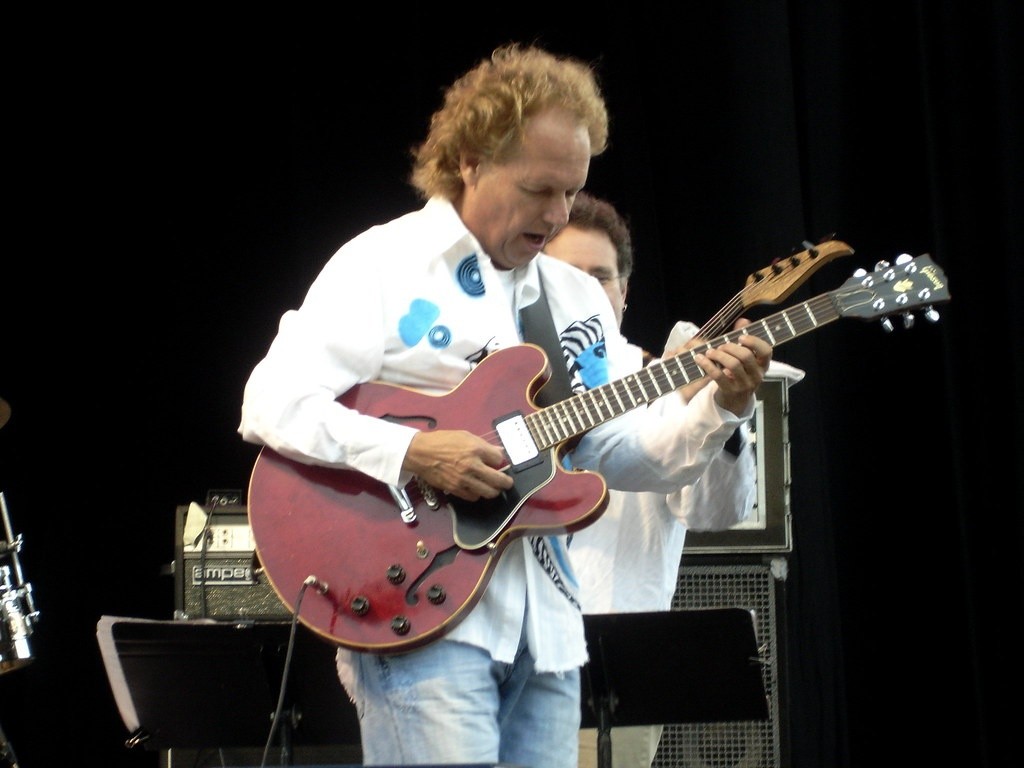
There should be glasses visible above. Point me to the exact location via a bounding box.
[595,271,626,284]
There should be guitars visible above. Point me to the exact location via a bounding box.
[678,236,858,355]
[245,250,954,656]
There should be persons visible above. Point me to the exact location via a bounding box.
[525,196,751,768]
[238,39,775,768]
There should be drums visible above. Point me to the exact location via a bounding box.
[0,564,36,676]
